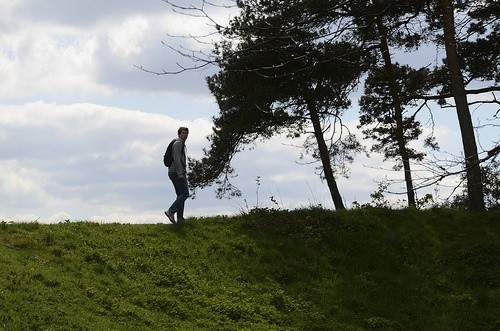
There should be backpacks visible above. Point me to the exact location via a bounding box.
[164,138,185,167]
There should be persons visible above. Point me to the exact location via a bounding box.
[165,127,190,224]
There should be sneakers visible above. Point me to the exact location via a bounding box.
[165,210,177,224]
